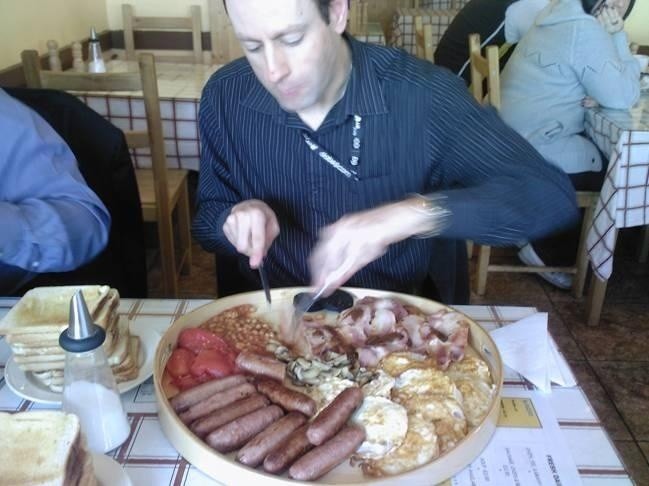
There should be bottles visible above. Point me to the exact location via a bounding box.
[59,289,133,455]
[71,38,84,72]
[47,39,62,73]
[85,25,105,73]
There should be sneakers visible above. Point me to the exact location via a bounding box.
[517,237,576,291]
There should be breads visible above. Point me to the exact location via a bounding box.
[0,284,140,391]
[0,410,97,485]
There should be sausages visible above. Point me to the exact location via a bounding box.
[167,351,366,481]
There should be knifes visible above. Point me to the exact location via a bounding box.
[255,256,273,302]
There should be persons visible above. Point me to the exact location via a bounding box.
[432,0,540,97]
[495,0,640,292]
[193,0,585,310]
[0,85,114,298]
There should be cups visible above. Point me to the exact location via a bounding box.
[633,53,649,71]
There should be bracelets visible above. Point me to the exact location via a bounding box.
[406,187,447,238]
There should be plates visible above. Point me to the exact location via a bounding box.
[639,72,649,89]
[3,324,162,402]
[87,448,133,486]
